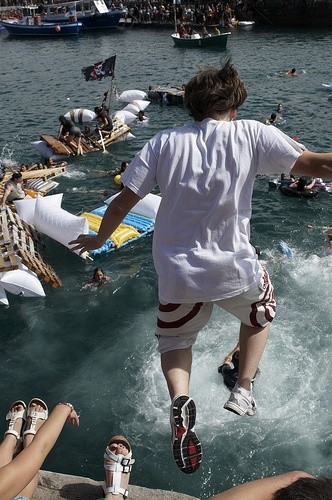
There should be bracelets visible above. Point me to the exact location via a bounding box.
[58,402,73,412]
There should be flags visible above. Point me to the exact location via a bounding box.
[65,6,75,17]
[82,55,115,81]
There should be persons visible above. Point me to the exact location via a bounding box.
[278,103,283,110]
[207,470,332,500]
[102,436,135,500]
[178,23,186,38]
[111,2,231,26]
[215,27,220,35]
[188,25,197,38]
[220,341,258,393]
[280,173,316,189]
[202,27,208,35]
[0,158,28,208]
[94,107,113,138]
[92,267,110,283]
[0,398,80,500]
[269,113,276,123]
[68,61,332,475]
[121,161,128,173]
[138,110,146,120]
[289,69,295,75]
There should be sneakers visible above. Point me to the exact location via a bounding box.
[170,394,203,475]
[223,381,257,416]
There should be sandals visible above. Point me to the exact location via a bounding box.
[3,400,27,453]
[103,435,135,499]
[23,398,48,441]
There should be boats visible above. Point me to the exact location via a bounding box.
[0,0,126,37]
[170,31,231,50]
